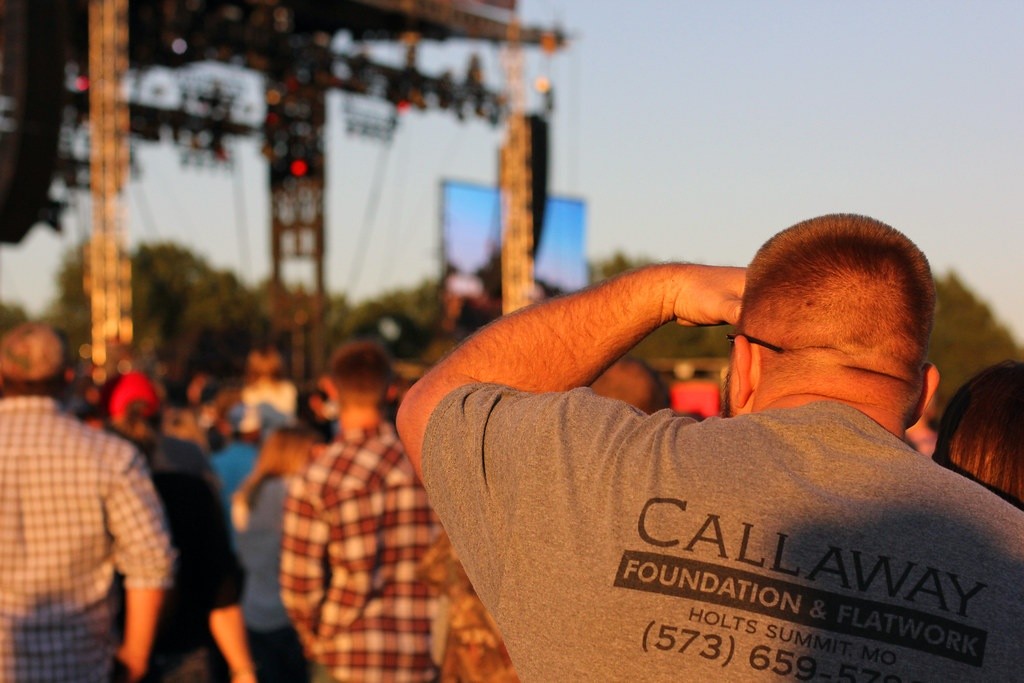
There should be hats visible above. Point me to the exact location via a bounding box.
[0,325,65,380]
[111,372,160,424]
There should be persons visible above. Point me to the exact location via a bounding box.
[0,321,1024,683]
[396,213,1024,683]
